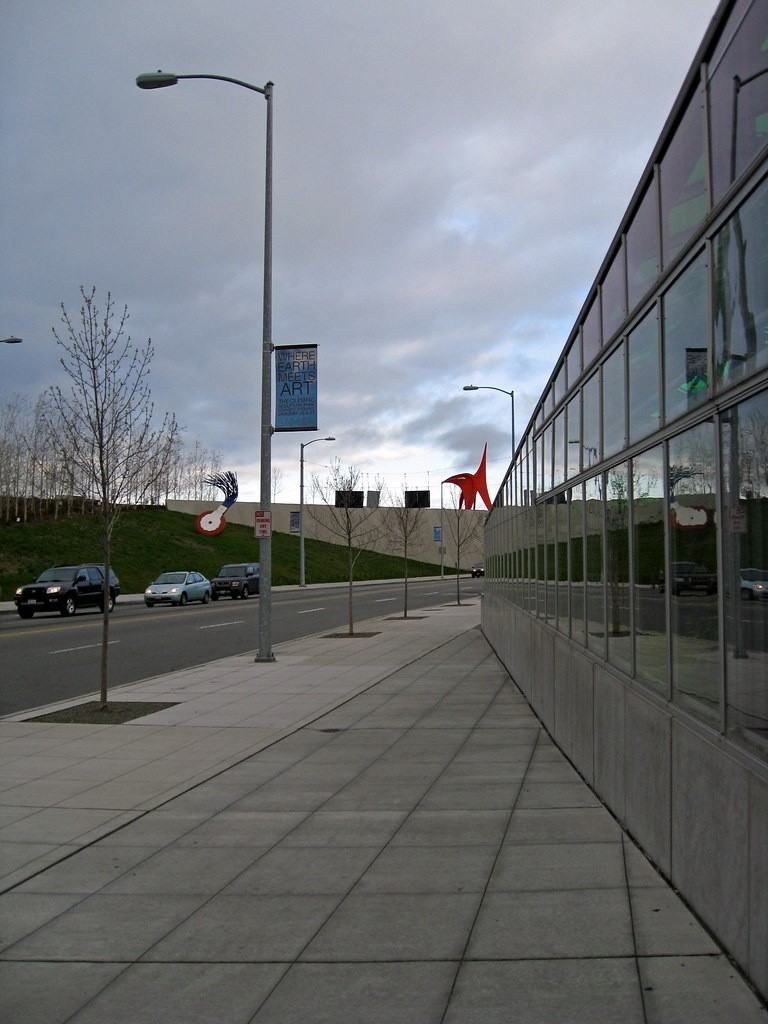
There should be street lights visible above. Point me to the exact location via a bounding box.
[133,70,275,666]
[569,438,592,470]
[300,436,336,585]
[463,385,516,456]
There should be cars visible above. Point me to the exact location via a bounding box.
[143,571,213,608]
[738,567,767,598]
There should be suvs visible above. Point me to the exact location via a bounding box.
[210,563,260,601]
[13,562,121,619]
[659,561,717,597]
[472,563,484,578]
